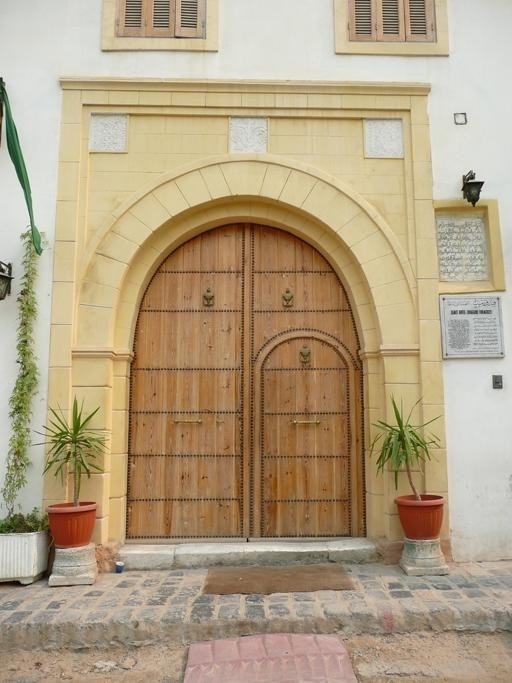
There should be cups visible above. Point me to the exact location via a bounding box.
[115,561,125,573]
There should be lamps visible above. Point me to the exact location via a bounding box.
[0,261,15,301]
[461,170,484,207]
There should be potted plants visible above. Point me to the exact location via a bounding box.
[365,391,447,540]
[28,395,111,548]
[0,224,49,586]
